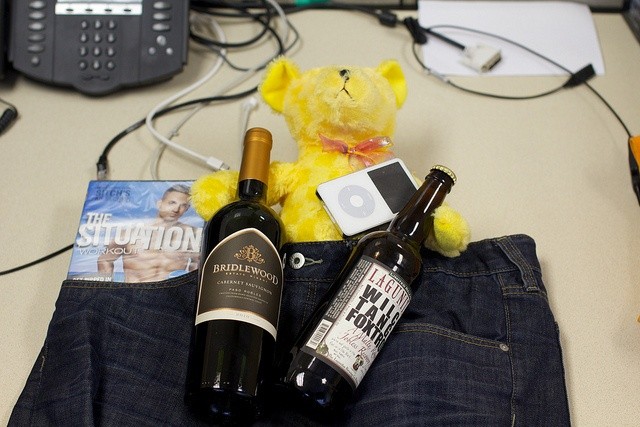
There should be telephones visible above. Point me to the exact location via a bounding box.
[0,0,191,99]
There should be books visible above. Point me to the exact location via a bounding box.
[66,180,205,281]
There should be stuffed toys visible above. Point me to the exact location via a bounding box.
[187,54,472,258]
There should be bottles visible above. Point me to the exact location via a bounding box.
[258,162,457,422]
[183,126,285,426]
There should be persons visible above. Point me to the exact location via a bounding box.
[96,185,199,282]
